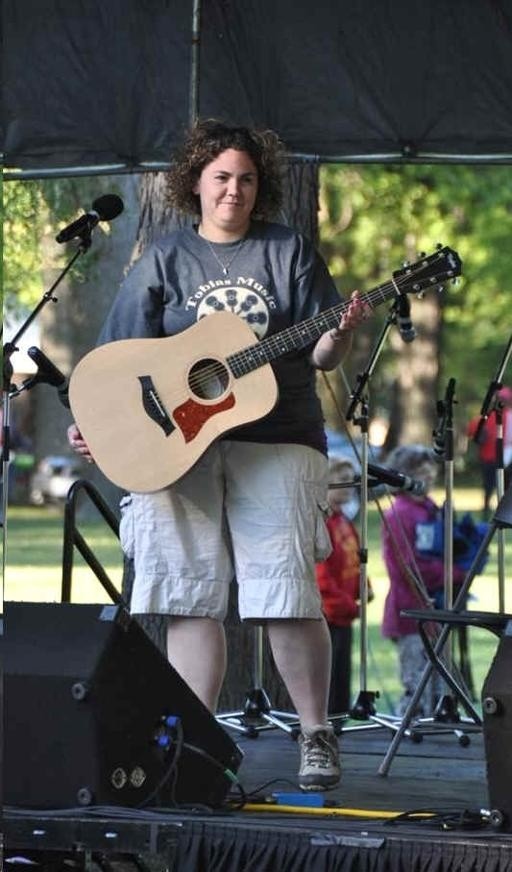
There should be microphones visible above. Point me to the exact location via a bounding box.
[365,462,429,497]
[54,191,127,247]
[395,287,420,347]
[431,374,461,466]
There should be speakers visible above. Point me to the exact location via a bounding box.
[1,598,245,814]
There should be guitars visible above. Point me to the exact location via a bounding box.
[68,243,462,495]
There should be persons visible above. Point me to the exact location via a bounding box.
[467,383,512,511]
[381,444,464,721]
[67,116,373,792]
[316,459,375,723]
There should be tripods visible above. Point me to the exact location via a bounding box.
[388,461,472,747]
[216,624,301,738]
[325,479,424,743]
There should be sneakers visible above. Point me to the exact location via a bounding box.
[297,721,340,790]
